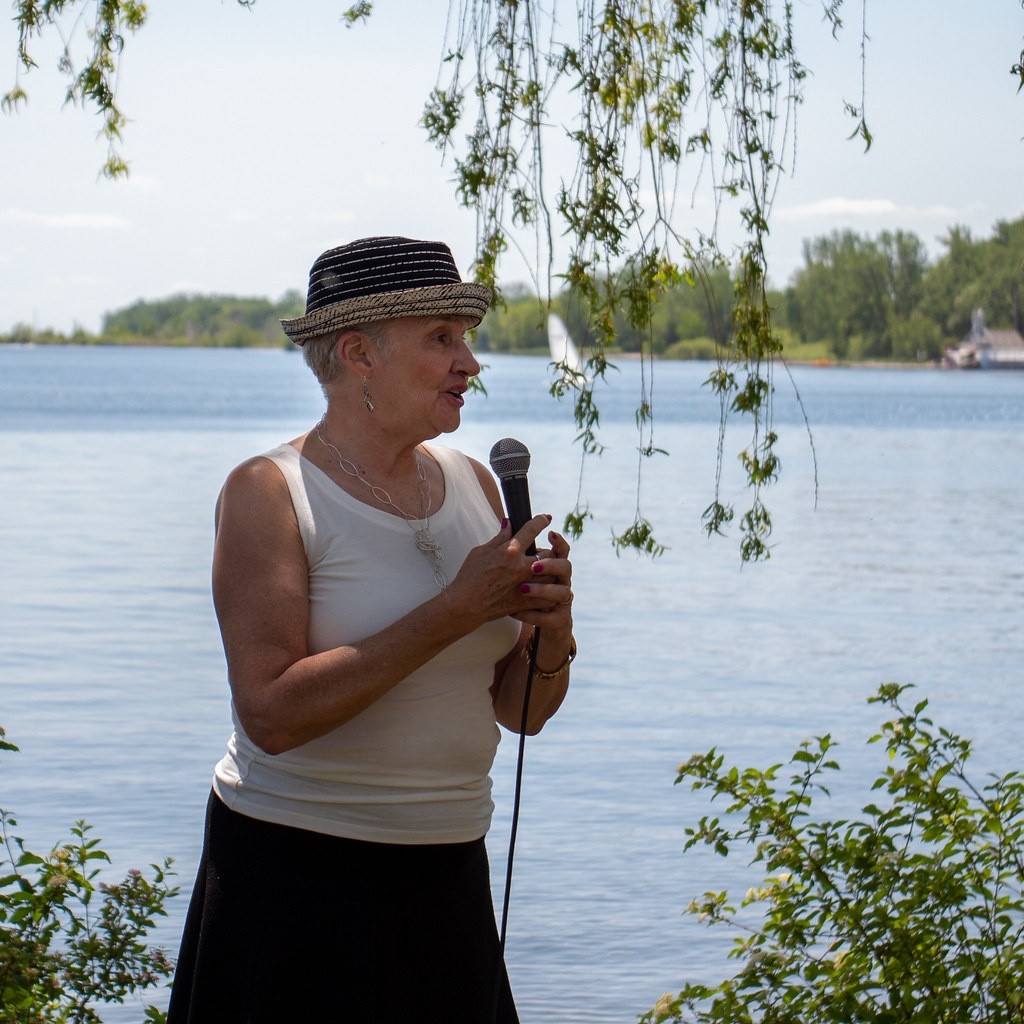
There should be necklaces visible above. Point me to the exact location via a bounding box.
[317,407,452,590]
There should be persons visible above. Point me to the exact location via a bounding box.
[162,231,583,1024]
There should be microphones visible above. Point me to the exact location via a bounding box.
[489,438,537,556]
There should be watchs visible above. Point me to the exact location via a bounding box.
[521,630,578,681]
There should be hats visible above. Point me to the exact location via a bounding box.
[280,236,493,345]
